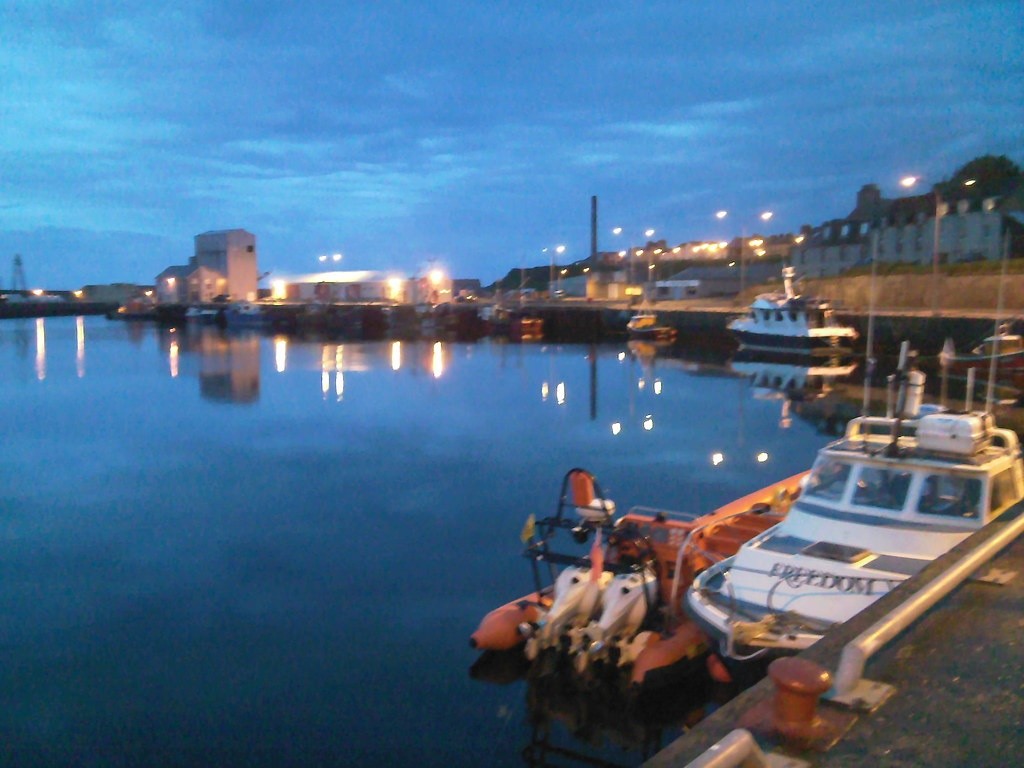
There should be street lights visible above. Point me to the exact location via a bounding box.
[542,245,564,285]
[899,176,977,283]
[612,227,655,285]
[715,210,774,290]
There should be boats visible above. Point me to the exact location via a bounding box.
[724,344,858,428]
[466,199,1023,709]
[625,295,677,341]
[726,266,859,357]
